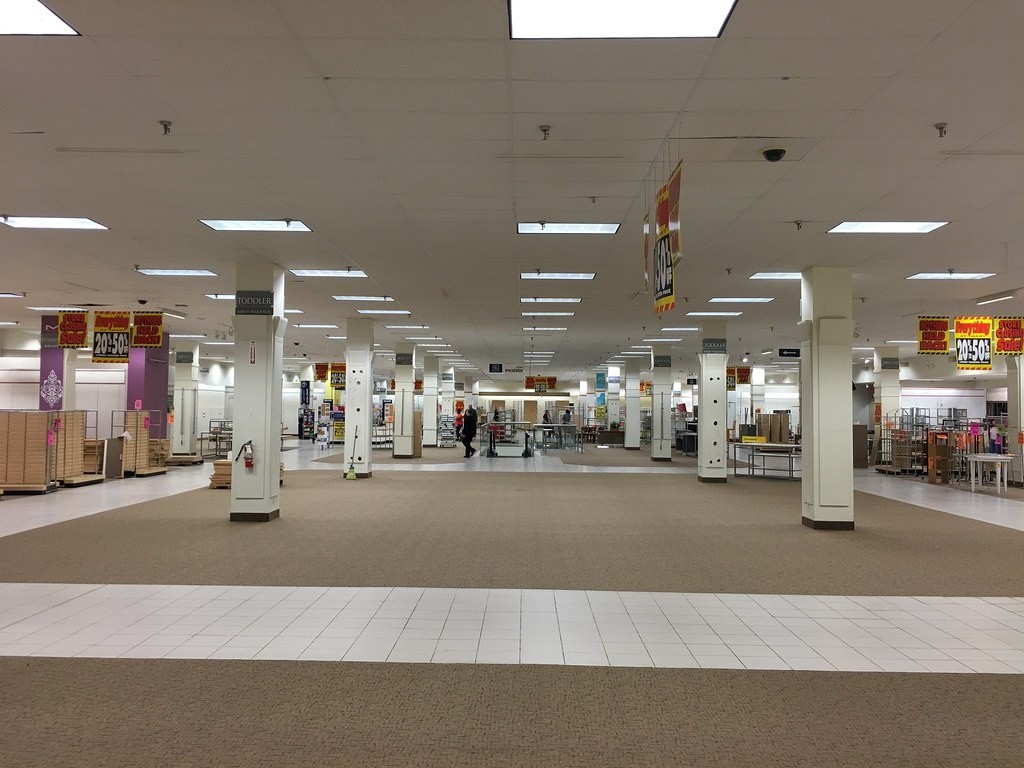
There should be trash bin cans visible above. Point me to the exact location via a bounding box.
[675,421,698,452]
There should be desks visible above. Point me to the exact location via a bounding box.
[596,430,625,446]
[734,443,802,481]
[967,453,1013,494]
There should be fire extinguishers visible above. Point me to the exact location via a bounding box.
[234,439,255,467]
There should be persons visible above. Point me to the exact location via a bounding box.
[493,408,499,421]
[455,404,486,442]
[562,410,571,424]
[461,410,476,459]
[983,422,998,453]
[542,410,554,438]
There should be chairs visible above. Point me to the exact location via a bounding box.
[575,426,597,443]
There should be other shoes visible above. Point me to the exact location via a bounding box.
[463,455,470,458]
[471,449,476,457]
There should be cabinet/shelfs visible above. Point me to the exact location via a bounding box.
[928,431,950,485]
[298,408,315,439]
[747,452,801,476]
